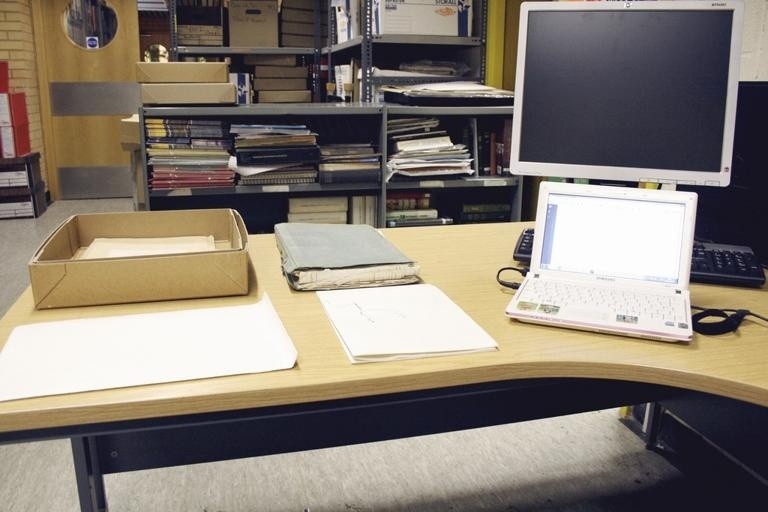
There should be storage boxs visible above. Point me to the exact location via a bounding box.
[28,206,251,310]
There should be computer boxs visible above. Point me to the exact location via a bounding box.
[589,81,768,269]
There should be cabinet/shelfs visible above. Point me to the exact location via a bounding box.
[0,90,46,220]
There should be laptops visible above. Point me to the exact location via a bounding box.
[504,181,698,344]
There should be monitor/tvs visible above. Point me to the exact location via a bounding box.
[510,2,745,188]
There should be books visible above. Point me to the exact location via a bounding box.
[287,197,377,227]
[386,190,512,226]
[271,220,422,292]
[387,116,513,182]
[145,119,381,188]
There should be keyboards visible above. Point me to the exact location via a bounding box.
[513,227,767,289]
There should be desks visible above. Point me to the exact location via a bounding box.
[0,222,768,512]
